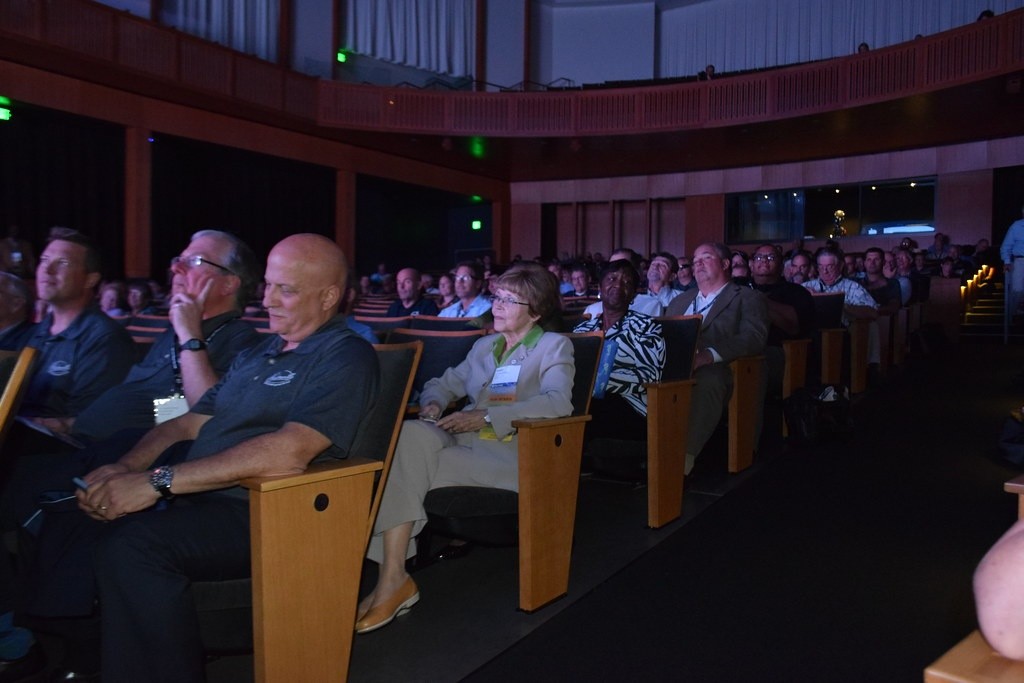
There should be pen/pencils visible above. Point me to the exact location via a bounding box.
[72,477,86,490]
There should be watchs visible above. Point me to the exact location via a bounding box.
[482,409,492,428]
[149,464,176,500]
[177,338,206,351]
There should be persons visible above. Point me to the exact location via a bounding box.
[972,518,1024,663]
[71,235,382,683]
[699,65,715,81]
[0,219,1024,636]
[915,34,923,40]
[858,42,869,53]
[977,10,994,22]
[0,227,261,683]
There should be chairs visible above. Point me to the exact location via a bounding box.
[0,255,1024,683]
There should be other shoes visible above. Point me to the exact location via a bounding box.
[354,575,420,633]
[49,666,99,682]
[683,474,689,494]
[0,638,48,678]
[580,455,594,476]
[435,538,474,560]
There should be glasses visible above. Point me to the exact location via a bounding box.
[817,262,843,273]
[490,295,529,307]
[754,254,777,262]
[170,254,243,282]
[452,273,480,283]
[678,263,691,270]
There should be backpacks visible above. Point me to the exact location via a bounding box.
[782,386,832,447]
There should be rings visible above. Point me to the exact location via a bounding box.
[96,504,107,511]
